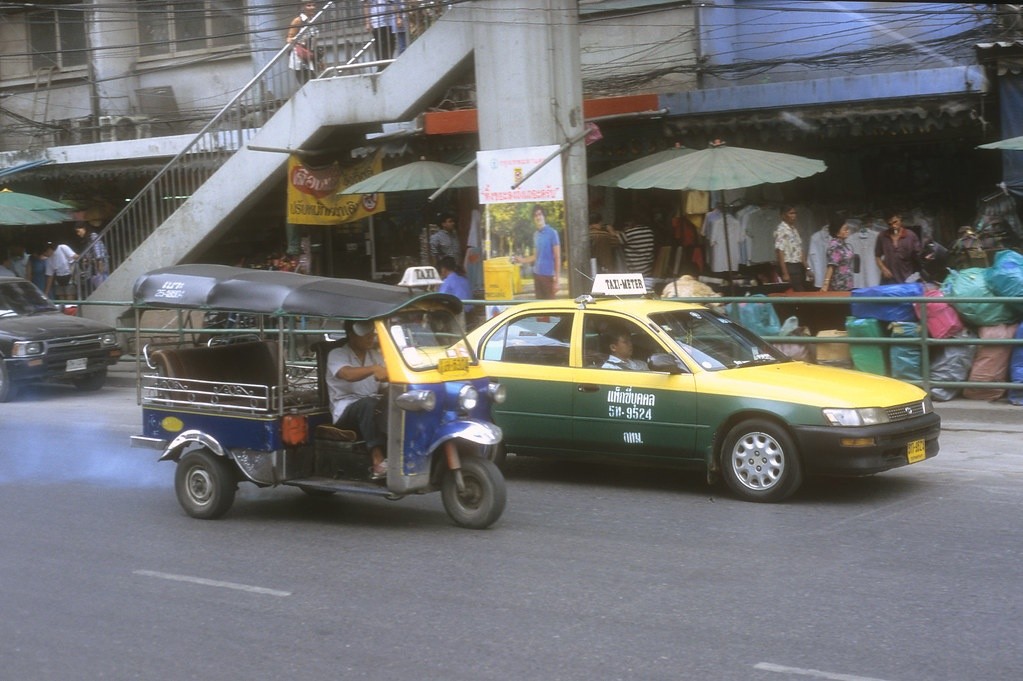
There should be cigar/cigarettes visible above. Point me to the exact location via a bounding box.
[894,230,897,234]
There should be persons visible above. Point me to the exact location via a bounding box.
[511,204,560,299]
[600,326,650,371]
[589,212,654,276]
[287,0,326,84]
[771,208,816,336]
[803,219,853,363]
[0,221,109,303]
[362,0,409,75]
[437,256,474,323]
[875,207,921,286]
[326,320,389,480]
[430,215,462,271]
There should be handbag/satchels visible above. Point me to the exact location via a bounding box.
[55,275,72,285]
[853,253,860,273]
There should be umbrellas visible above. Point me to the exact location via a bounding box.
[609,133,827,298]
[588,141,699,273]
[975,136,1023,150]
[335,154,477,240]
[0,188,73,224]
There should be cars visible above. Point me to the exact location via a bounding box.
[415,272,942,506]
[0,276,123,404]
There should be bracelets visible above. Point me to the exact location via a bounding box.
[824,279,829,280]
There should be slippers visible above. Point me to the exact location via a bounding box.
[372,462,388,479]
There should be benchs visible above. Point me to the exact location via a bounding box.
[505,347,571,368]
[157,341,307,411]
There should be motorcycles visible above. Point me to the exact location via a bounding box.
[130,261,508,531]
